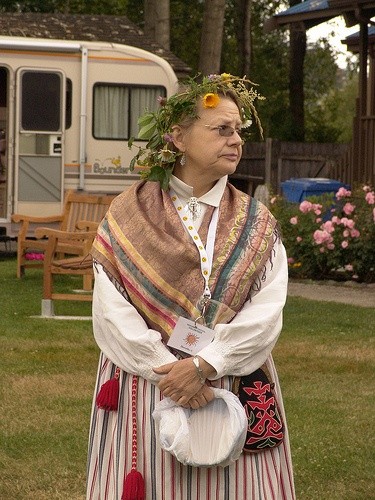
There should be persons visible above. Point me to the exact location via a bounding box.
[85,73,296,500]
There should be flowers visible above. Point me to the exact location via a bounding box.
[127,74,265,194]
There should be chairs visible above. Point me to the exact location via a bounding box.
[35,220,101,319]
[10,191,120,279]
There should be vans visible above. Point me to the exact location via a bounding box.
[0,35,179,240]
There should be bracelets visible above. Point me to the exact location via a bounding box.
[193,355,204,385]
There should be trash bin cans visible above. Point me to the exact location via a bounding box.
[279,177,351,261]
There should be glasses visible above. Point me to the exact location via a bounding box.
[197,119,246,139]
